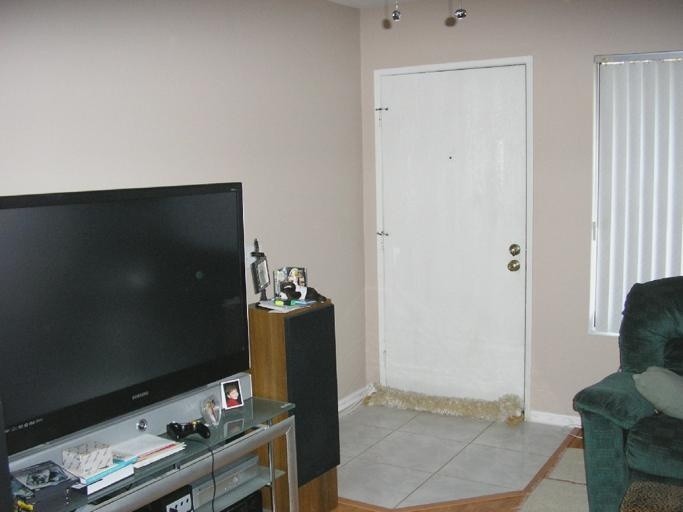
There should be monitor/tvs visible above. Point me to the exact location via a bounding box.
[0,179,251,476]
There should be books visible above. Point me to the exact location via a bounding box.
[63,431,188,499]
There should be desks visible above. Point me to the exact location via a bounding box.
[10,397,298,512]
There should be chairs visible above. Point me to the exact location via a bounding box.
[572,275,683,512]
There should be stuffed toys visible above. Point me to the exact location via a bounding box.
[278,281,327,303]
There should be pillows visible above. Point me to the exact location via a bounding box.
[631,365,683,420]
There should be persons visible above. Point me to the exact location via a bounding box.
[223,381,243,408]
